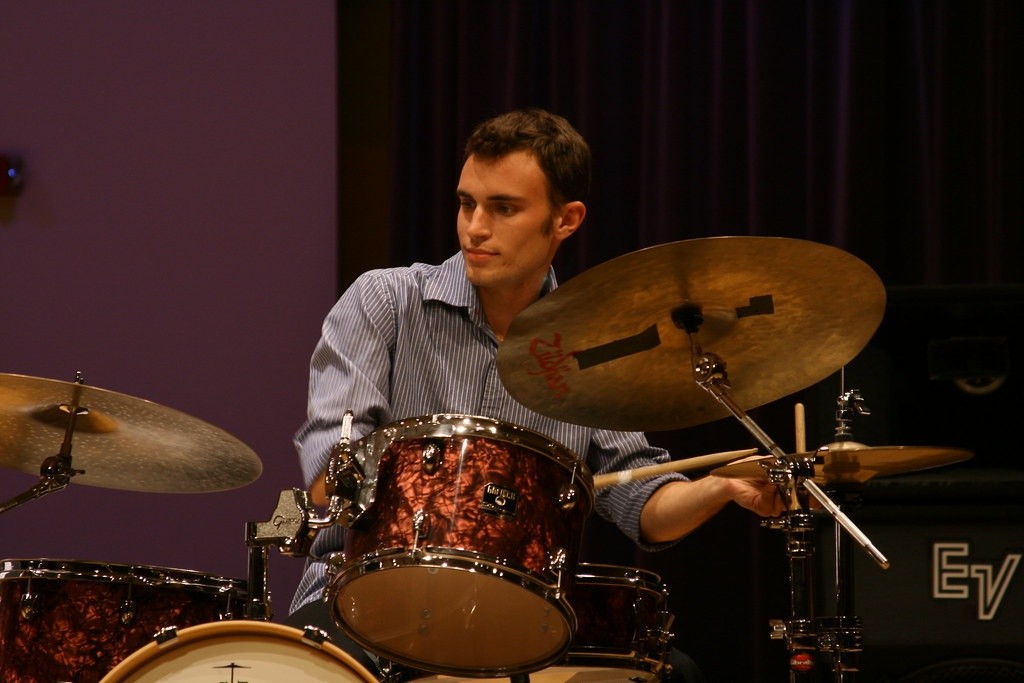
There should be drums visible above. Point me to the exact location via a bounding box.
[0,556,273,683]
[324,410,599,677]
[97,620,383,682]
[560,559,674,672]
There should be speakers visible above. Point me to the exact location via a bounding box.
[814,503,1024,683]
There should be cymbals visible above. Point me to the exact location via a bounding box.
[0,372,263,496]
[494,235,889,433]
[711,444,978,492]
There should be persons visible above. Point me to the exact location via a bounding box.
[288,112,822,683]
